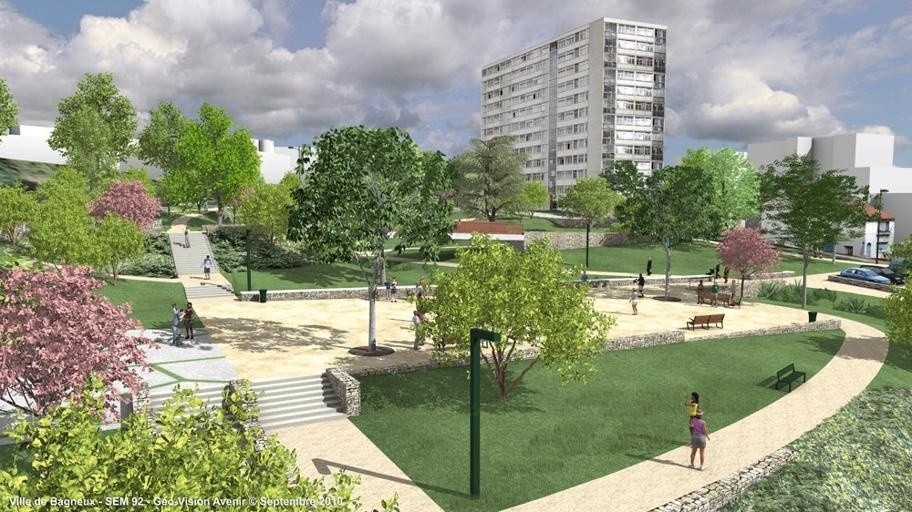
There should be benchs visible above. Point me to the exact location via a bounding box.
[687,313,725,331]
[776,363,806,392]
